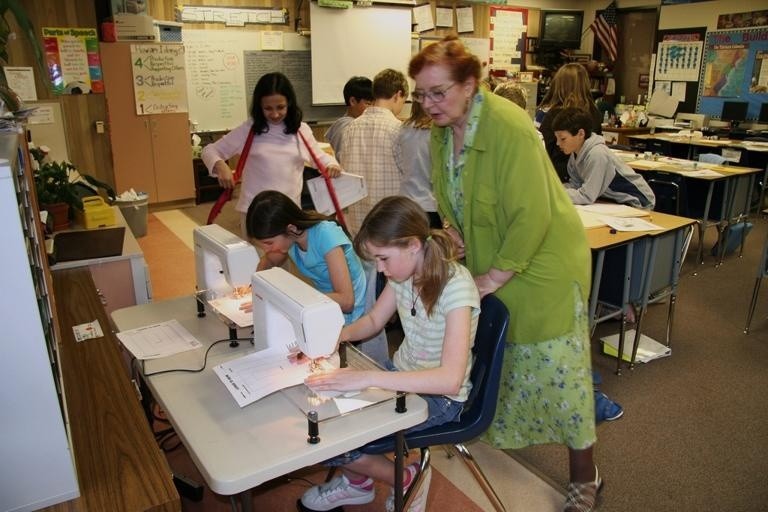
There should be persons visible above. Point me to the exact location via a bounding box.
[201,72,343,274]
[551,107,656,324]
[493,82,545,149]
[287,196,483,512]
[323,76,374,153]
[339,69,409,329]
[394,93,443,229]
[233,190,367,348]
[536,62,603,183]
[407,34,604,512]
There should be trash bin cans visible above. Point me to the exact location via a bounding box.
[112,194,149,238]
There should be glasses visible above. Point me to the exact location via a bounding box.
[411,82,458,103]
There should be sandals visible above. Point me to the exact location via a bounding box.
[561,464,603,512]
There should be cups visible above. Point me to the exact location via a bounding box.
[644,152,653,161]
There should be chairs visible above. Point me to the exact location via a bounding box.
[357,294,511,512]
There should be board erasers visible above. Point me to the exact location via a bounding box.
[306,121,318,125]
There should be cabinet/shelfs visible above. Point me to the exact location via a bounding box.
[192,158,230,206]
[99,40,196,212]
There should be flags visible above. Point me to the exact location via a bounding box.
[589,2,620,63]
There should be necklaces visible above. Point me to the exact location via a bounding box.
[411,281,423,316]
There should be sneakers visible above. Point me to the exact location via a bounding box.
[387,458,432,512]
[302,475,375,511]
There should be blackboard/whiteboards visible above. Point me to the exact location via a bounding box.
[177,31,349,139]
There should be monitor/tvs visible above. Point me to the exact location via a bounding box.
[721,102,749,130]
[758,102,768,125]
[537,9,584,50]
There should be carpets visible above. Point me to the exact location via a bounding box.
[178,184,767,511]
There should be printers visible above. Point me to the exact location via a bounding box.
[674,113,710,132]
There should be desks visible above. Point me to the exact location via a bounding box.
[744,209,767,335]
[573,202,697,375]
[110,293,429,511]
[600,126,767,268]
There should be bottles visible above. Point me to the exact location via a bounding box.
[603,105,655,134]
